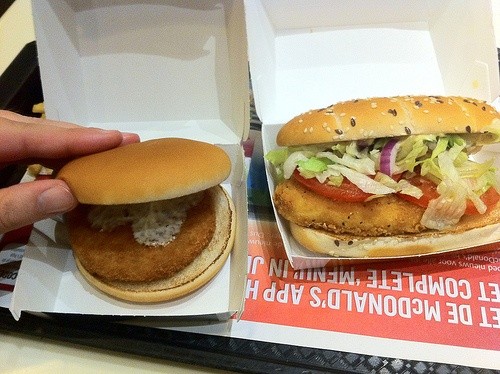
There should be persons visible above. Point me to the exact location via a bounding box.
[0,108,142,236]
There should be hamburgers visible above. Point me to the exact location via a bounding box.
[54,137,237,304]
[264,94,500,257]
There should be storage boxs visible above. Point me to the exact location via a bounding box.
[9,0,252,324]
[239,2,500,270]
